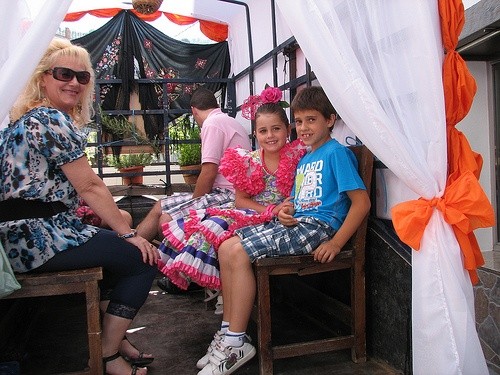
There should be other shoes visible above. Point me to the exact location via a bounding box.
[204,287,219,302]
[158,276,203,295]
[213,295,224,314]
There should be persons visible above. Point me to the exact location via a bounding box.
[195,85,372,375]
[135,88,253,242]
[77,194,134,232]
[155,102,312,314]
[0,35,162,375]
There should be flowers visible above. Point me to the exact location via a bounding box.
[260,83,291,109]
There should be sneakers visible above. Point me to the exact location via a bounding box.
[196,331,226,369]
[197,340,256,375]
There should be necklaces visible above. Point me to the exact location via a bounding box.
[43,99,53,108]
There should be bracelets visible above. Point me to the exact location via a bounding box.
[117,229,138,239]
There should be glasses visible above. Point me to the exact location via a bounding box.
[46,67,91,84]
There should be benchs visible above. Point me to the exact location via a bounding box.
[201,145,374,375]
[1,266,107,375]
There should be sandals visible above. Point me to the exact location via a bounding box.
[88,352,148,375]
[118,335,153,366]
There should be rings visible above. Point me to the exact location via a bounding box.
[150,244,153,249]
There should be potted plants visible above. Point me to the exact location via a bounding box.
[169,118,202,184]
[106,151,155,185]
